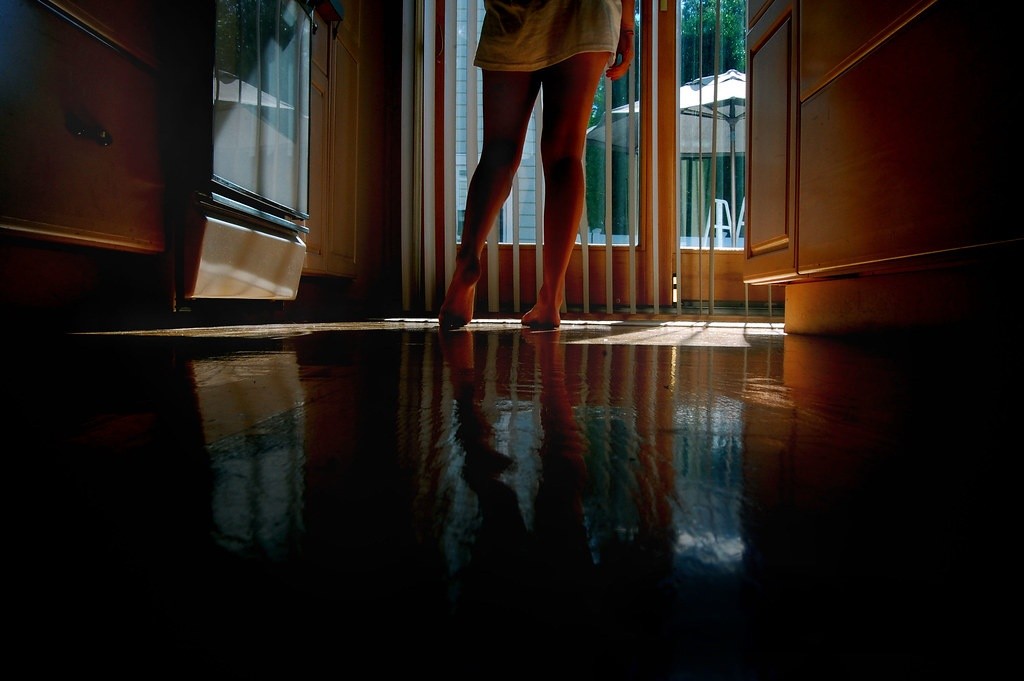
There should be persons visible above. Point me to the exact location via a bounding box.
[438,0,635,330]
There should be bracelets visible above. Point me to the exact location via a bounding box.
[620,29,634,35]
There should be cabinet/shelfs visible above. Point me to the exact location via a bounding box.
[743,0,1024,334]
[296,0,400,320]
[0,0,185,318]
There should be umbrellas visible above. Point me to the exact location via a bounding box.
[586,69,746,157]
